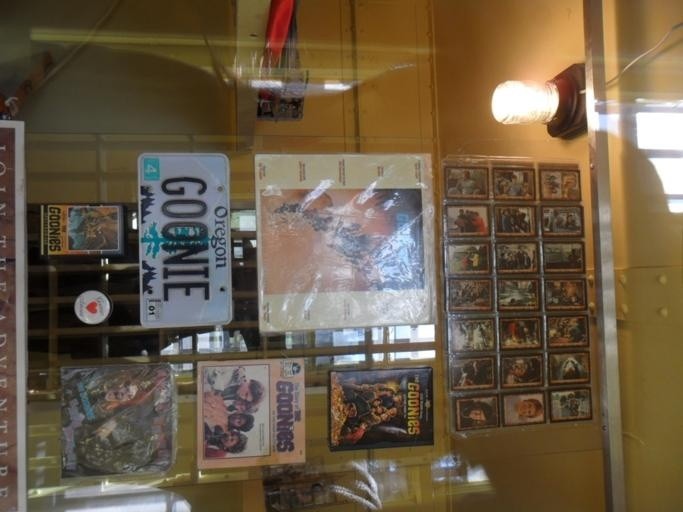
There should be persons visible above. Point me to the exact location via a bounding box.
[340,375,404,445]
[446,170,590,426]
[202,365,265,454]
[88,371,142,416]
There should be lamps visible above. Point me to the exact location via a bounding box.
[488,63,587,139]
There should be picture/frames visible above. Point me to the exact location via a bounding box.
[496,278,542,312]
[542,241,585,275]
[546,351,590,386]
[544,278,587,311]
[500,391,547,427]
[449,357,498,391]
[444,166,489,200]
[548,387,593,423]
[498,317,543,350]
[538,168,581,201]
[444,240,492,277]
[540,205,584,239]
[494,241,540,275]
[545,316,589,348]
[493,204,538,238]
[500,354,544,388]
[452,396,499,430]
[447,278,494,312]
[444,204,490,238]
[492,166,536,201]
[449,318,496,352]
